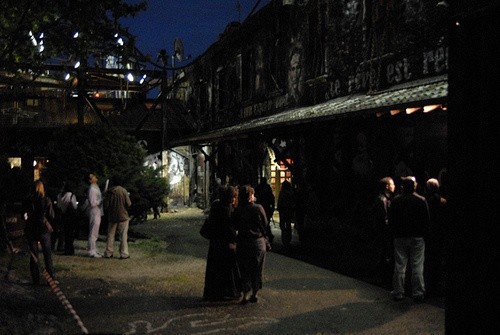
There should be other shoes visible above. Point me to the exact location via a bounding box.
[394,293,404,299]
[91,251,104,258]
[413,296,424,302]
[105,253,113,258]
[242,294,258,304]
[119,256,130,259]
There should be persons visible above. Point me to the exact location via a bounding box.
[141,200,168,223]
[51,173,132,259]
[19,180,63,288]
[375,175,448,303]
[199,177,296,305]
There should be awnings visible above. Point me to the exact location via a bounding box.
[164,80,448,145]
